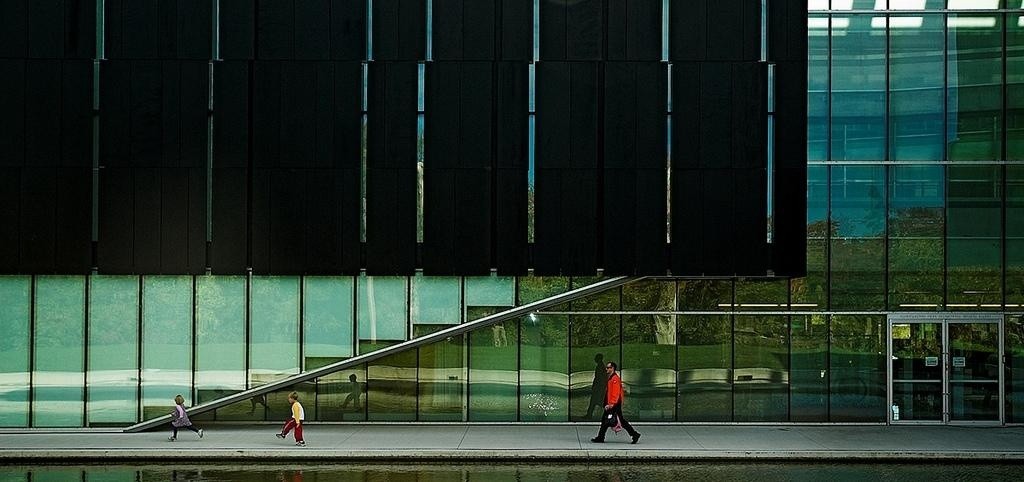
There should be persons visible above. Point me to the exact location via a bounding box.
[580,353,611,422]
[167,394,204,442]
[272,389,306,447]
[591,361,642,445]
[338,373,366,408]
[245,395,270,417]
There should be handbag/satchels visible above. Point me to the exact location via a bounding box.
[605,408,622,432]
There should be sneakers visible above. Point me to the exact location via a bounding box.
[167,436,177,441]
[630,432,641,444]
[276,434,285,440]
[197,428,203,438]
[590,436,604,443]
[294,440,306,447]
